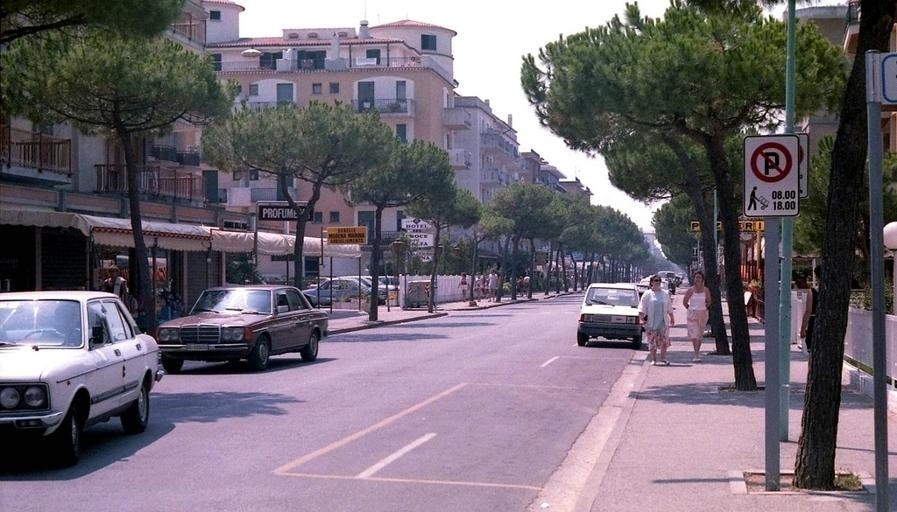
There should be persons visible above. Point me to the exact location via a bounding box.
[638,273,675,367]
[682,270,712,360]
[799,264,821,353]
[102,265,130,312]
[457,271,531,302]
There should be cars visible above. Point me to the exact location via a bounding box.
[638,271,685,298]
[155,284,329,371]
[0,291,164,466]
[576,283,642,349]
[302,275,399,308]
